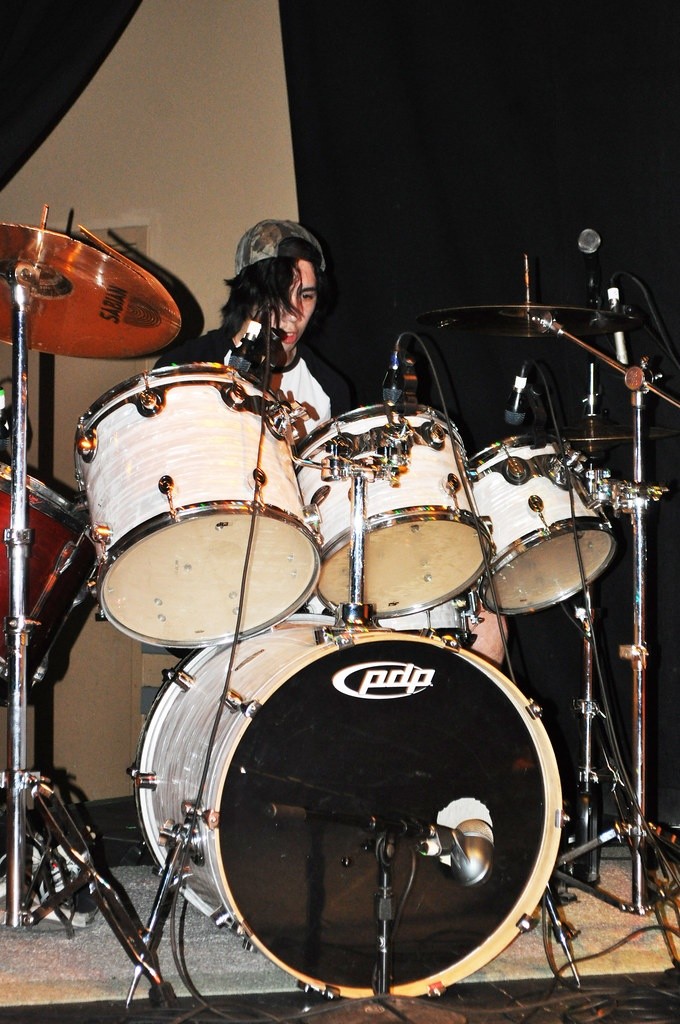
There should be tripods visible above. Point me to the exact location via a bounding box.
[0,280,185,1016]
[528,310,680,916]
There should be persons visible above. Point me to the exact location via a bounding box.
[155,219,508,666]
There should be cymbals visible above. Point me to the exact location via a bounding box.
[416,300,639,339]
[0,222,182,359]
[539,417,678,458]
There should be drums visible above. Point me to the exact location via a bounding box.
[0,458,104,689]
[73,361,322,650]
[292,403,491,621]
[307,589,469,640]
[467,431,618,616]
[132,614,567,1001]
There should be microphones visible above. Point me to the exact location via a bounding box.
[607,278,628,365]
[229,307,266,372]
[505,365,530,425]
[420,819,495,886]
[578,228,603,308]
[382,348,405,404]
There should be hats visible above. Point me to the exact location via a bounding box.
[234,218,326,274]
